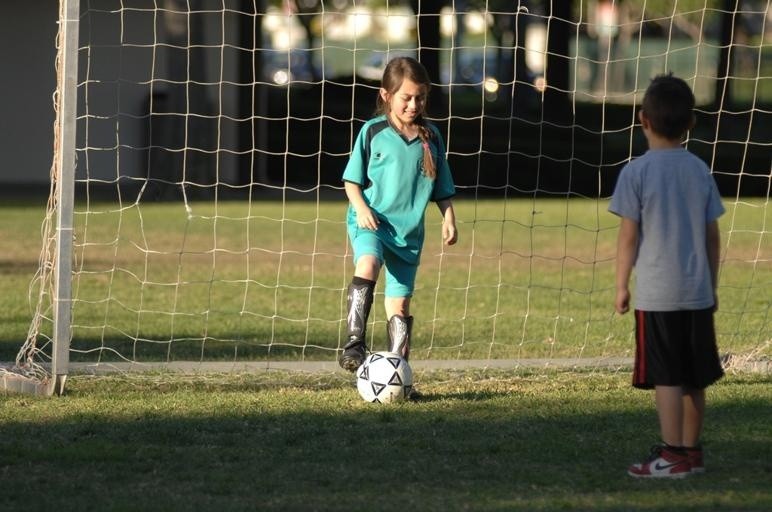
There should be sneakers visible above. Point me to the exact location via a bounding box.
[626,437,706,479]
[339,339,366,371]
[407,387,424,402]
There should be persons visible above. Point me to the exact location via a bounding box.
[607,68,729,481]
[336,54,460,397]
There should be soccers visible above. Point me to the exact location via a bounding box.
[356,351,414,405]
